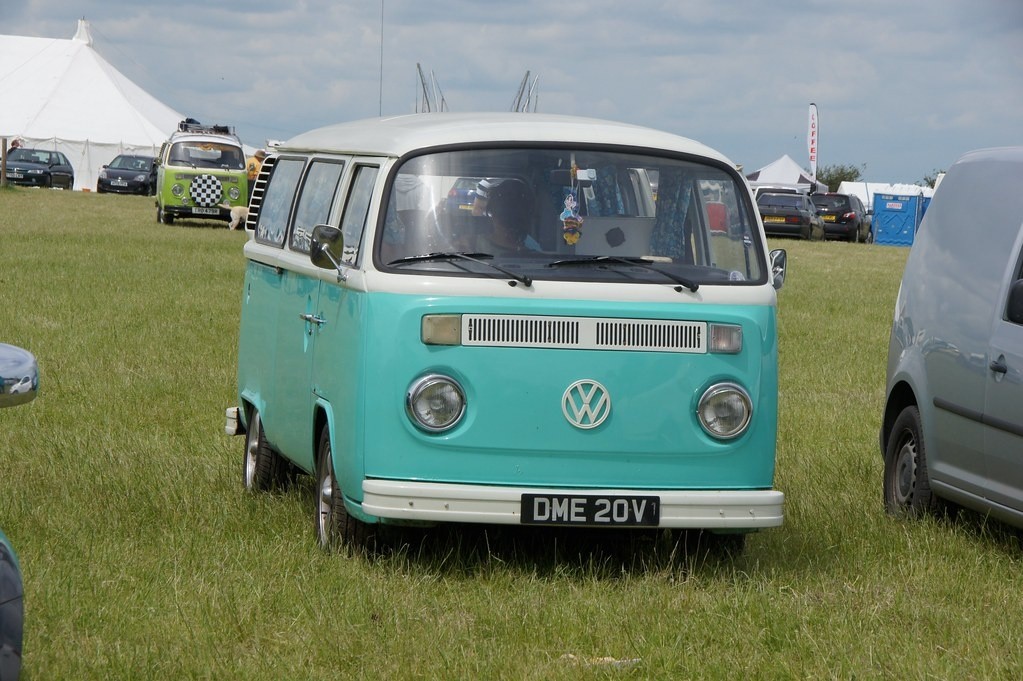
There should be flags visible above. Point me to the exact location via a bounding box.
[807,103,818,184]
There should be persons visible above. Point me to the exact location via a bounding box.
[8,140,19,154]
[380,174,570,262]
[246,150,265,198]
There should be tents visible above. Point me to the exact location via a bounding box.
[0,16,269,192]
[700,154,829,201]
[836,172,946,246]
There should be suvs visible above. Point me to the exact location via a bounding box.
[810,192,875,245]
[754,184,802,201]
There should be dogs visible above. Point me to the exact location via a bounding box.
[218,204,249,231]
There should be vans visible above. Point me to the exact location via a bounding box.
[649,179,732,238]
[152,122,255,231]
[222,109,789,557]
[876,143,1022,536]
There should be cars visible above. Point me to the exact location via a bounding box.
[0,148,75,191]
[0,340,42,681]
[9,374,33,394]
[95,152,159,197]
[446,177,488,219]
[755,193,829,242]
